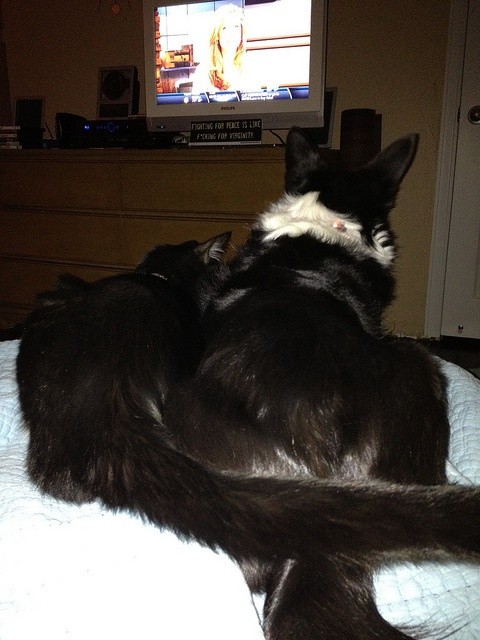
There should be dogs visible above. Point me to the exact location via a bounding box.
[169,125,449,640]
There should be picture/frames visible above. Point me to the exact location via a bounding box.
[11,95,46,138]
[310,86,338,149]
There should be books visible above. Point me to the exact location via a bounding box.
[0,126,22,151]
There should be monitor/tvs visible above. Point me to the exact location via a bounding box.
[142,0,328,146]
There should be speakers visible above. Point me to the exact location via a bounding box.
[96,65,140,120]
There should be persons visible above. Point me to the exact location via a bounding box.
[192,3,250,95]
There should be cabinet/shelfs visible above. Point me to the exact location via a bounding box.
[1,147,286,332]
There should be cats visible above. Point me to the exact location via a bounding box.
[14,230,480,565]
